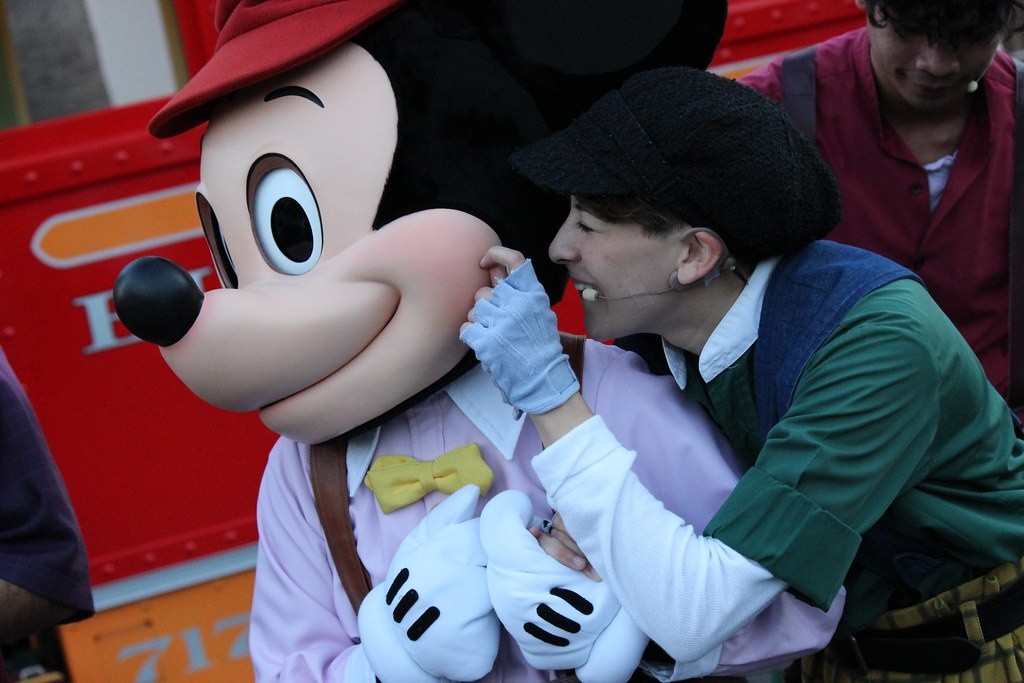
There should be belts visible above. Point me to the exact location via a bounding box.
[825,577,1022,677]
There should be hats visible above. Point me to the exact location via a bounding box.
[511,67,845,256]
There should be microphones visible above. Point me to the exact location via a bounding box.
[581,229,728,301]
[967,51,994,92]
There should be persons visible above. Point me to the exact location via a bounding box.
[0,341,98,649]
[731,0,1023,428]
[459,64,1024,683]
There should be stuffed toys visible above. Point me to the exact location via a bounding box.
[113,0,847,682]
